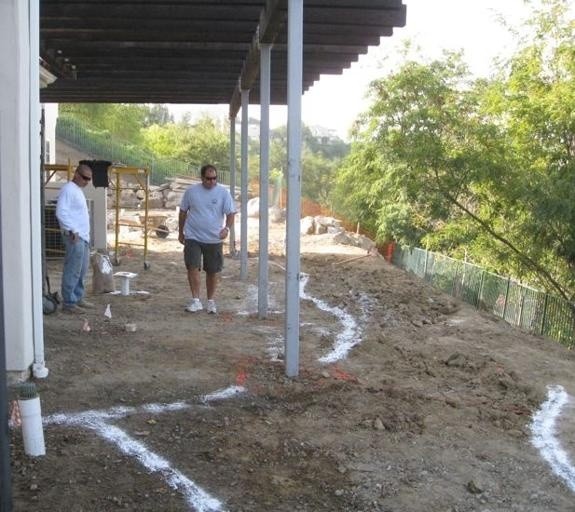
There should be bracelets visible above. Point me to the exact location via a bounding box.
[224,225,231,232]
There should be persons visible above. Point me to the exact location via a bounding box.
[53,165,98,318]
[176,164,238,316]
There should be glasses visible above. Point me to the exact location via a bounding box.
[77,170,91,181]
[203,176,217,181]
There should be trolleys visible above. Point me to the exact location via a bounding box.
[114,213,172,239]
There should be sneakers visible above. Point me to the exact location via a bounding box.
[206,300,217,314]
[183,301,204,313]
[61,303,86,315]
[77,300,95,309]
